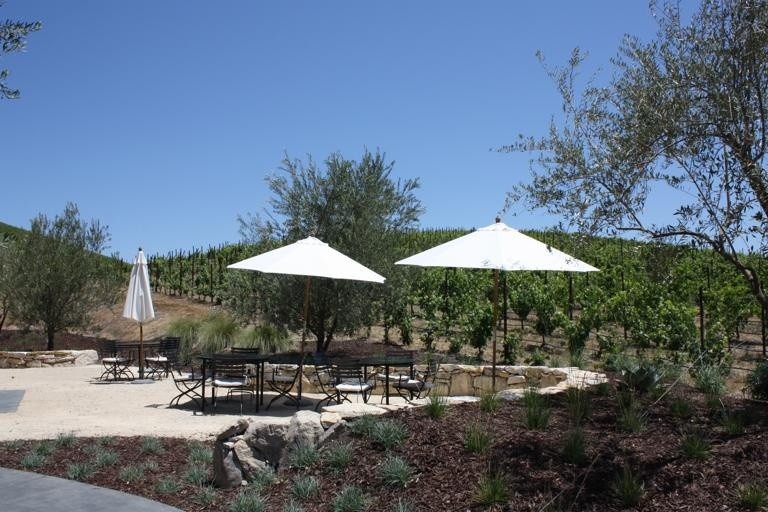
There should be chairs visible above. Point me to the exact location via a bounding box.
[93,335,182,382]
[167,346,443,414]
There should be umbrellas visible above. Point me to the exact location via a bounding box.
[123,247,155,380]
[226,236,387,399]
[394,215,601,393]
